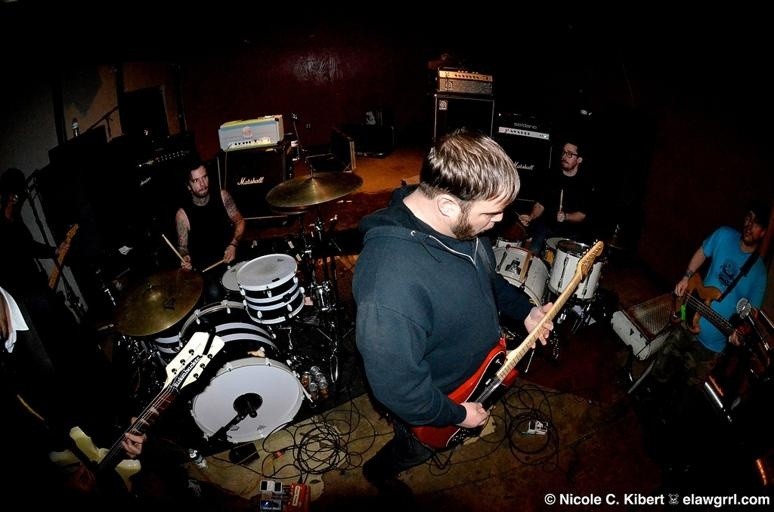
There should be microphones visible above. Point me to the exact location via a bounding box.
[246,398,257,419]
[11,175,34,204]
[736,298,770,351]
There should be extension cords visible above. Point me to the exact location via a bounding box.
[527,420,548,435]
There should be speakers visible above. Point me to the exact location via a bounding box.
[494,127,549,200]
[433,94,496,141]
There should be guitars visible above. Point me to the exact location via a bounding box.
[414,241,603,452]
[46,332,228,512]
[671,271,761,358]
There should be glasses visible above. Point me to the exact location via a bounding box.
[561,149,578,159]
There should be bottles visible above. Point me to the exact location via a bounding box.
[301,365,330,410]
[188,448,210,473]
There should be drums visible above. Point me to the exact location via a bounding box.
[221,260,244,301]
[236,253,305,326]
[178,299,305,443]
[492,246,550,306]
[495,237,523,248]
[543,238,571,271]
[154,331,180,358]
[547,240,608,302]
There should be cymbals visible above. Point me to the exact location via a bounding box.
[263,170,364,210]
[114,267,204,337]
[599,225,627,251]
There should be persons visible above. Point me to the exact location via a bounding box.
[0,167,77,343]
[175,158,246,302]
[353,128,554,503]
[0,288,148,510]
[518,134,594,257]
[674,198,770,392]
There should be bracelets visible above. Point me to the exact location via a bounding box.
[229,242,238,248]
[684,270,694,279]
[565,213,568,220]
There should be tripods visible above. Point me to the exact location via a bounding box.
[294,218,356,396]
